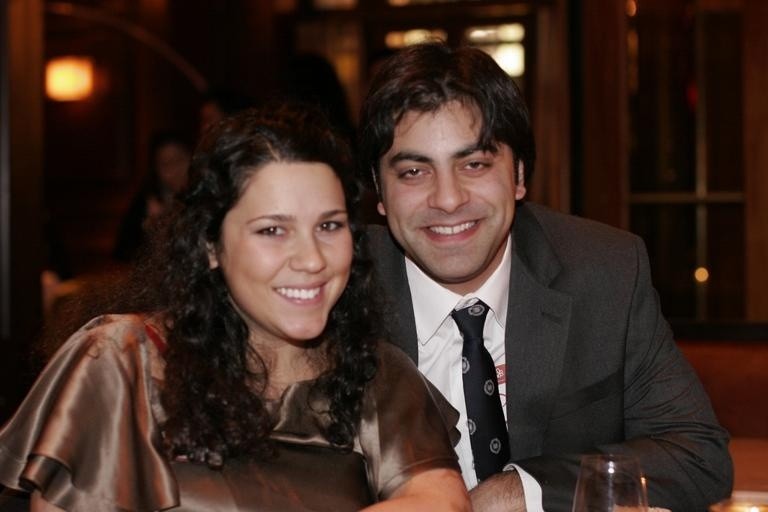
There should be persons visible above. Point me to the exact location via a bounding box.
[352,40,734,511]
[0,99,475,512]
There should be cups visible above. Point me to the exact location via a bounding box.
[571,453,649,512]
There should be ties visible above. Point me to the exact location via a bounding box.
[451,298,511,485]
